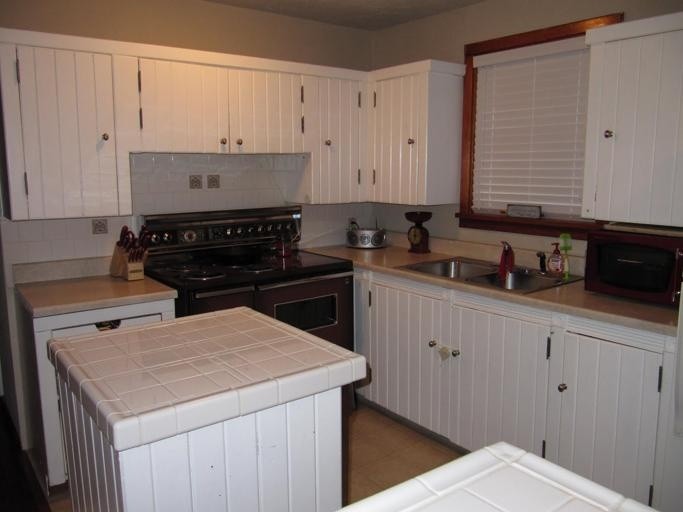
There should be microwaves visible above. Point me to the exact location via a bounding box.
[583,228,683,306]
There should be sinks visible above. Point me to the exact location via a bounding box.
[466,271,585,295]
[399,256,498,280]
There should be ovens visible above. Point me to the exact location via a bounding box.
[178,268,354,352]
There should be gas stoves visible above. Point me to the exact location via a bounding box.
[144,239,352,289]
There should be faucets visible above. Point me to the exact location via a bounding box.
[500,239,513,263]
[547,242,564,274]
[536,251,546,275]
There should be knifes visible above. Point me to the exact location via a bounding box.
[115,221,148,262]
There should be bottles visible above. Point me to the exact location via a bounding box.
[275,228,292,257]
[350,219,359,230]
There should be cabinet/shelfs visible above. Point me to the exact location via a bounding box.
[580,12,683,227]
[302,62,370,204]
[15,299,175,487]
[0,28,132,222]
[370,60,465,207]
[119,41,302,215]
[353,270,550,458]
[539,312,683,512]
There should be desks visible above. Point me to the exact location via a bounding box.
[337,441,658,512]
[46,307,367,512]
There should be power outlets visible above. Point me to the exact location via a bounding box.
[92,218,108,234]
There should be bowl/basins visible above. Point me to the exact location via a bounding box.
[345,228,387,250]
